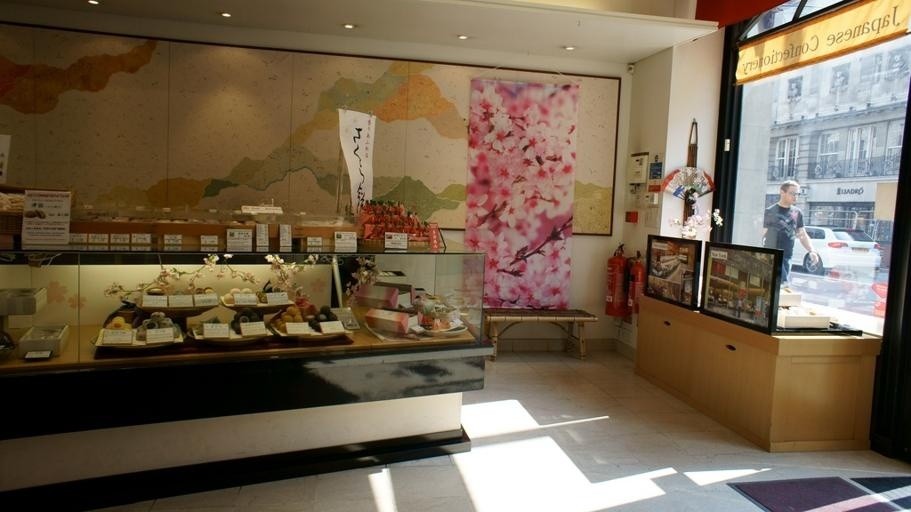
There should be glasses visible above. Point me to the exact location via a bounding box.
[786,189,801,197]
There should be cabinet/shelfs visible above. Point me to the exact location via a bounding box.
[1,236,496,512]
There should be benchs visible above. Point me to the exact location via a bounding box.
[482,307,600,362]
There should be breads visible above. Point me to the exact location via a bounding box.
[107,287,338,341]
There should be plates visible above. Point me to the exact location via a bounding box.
[91,293,351,346]
[416,322,468,337]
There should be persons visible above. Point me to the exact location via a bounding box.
[736,297,744,318]
[650,263,665,279]
[763,179,819,309]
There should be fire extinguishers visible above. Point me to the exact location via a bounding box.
[606,243,644,326]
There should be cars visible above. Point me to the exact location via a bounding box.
[763,226,881,274]
